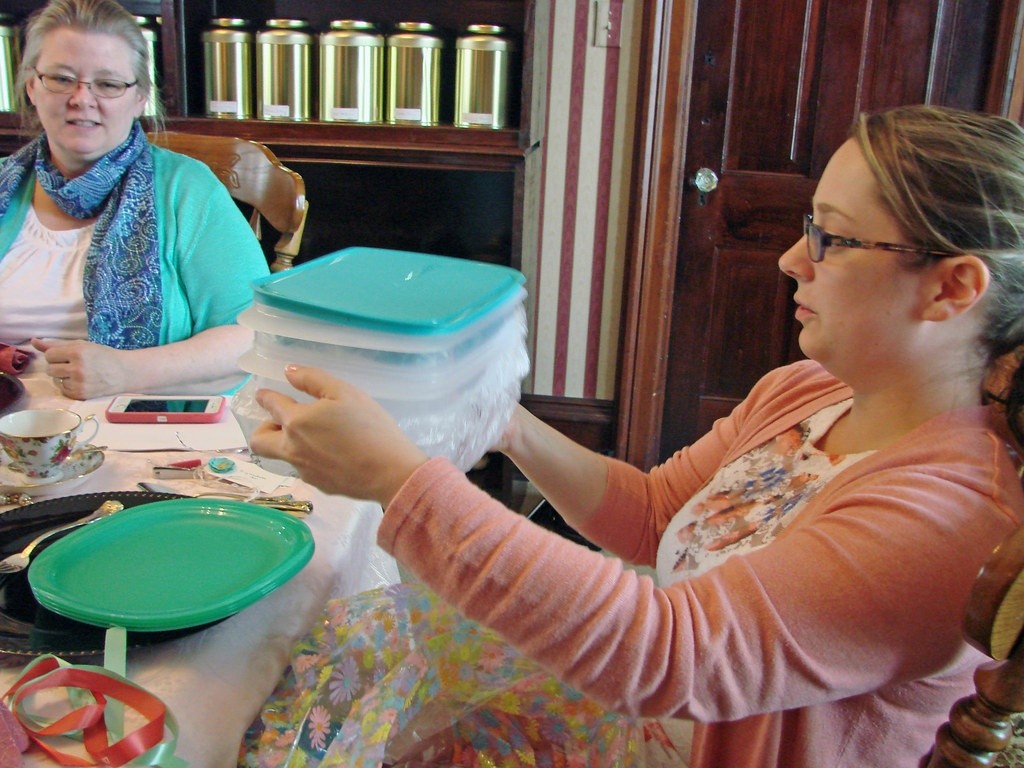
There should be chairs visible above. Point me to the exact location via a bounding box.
[143,131,310,273]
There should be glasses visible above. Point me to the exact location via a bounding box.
[33,65,137,99]
[803,215,959,264]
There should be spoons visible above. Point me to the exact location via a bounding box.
[138,483,293,502]
[8,446,108,470]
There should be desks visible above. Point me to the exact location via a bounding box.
[0,367,417,768]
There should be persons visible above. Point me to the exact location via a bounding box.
[0,0,269,404]
[249,107,1023,768]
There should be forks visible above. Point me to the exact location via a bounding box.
[0,501,124,573]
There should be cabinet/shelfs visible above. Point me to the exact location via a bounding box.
[0,0,536,272]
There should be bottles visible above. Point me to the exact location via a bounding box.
[1,14,27,112]
[256,19,312,122]
[386,22,444,126]
[319,20,384,124]
[131,14,163,117]
[202,18,253,120]
[454,24,513,129]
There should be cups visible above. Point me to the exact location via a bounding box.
[0,408,100,485]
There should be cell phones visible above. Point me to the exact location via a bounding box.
[108,394,227,425]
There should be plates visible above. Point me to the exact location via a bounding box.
[28,498,316,632]
[0,491,239,656]
[0,441,105,487]
